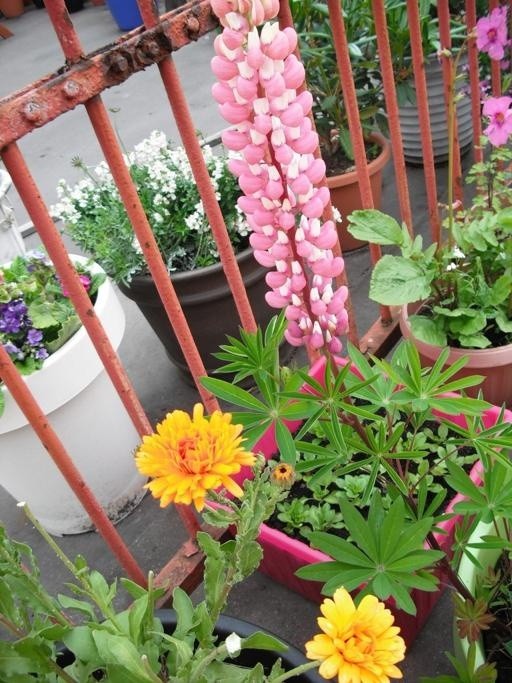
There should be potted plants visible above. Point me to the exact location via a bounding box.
[366,0,482,166]
[288,0,432,252]
[202,355,511,653]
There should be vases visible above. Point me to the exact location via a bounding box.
[115,234,297,391]
[454,516,512,683]
[400,264,512,411]
[0,255,151,534]
[27,609,335,683]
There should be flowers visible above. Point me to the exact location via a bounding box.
[0,402,408,683]
[198,0,512,683]
[48,129,342,288]
[346,6,512,349]
[0,244,106,418]
